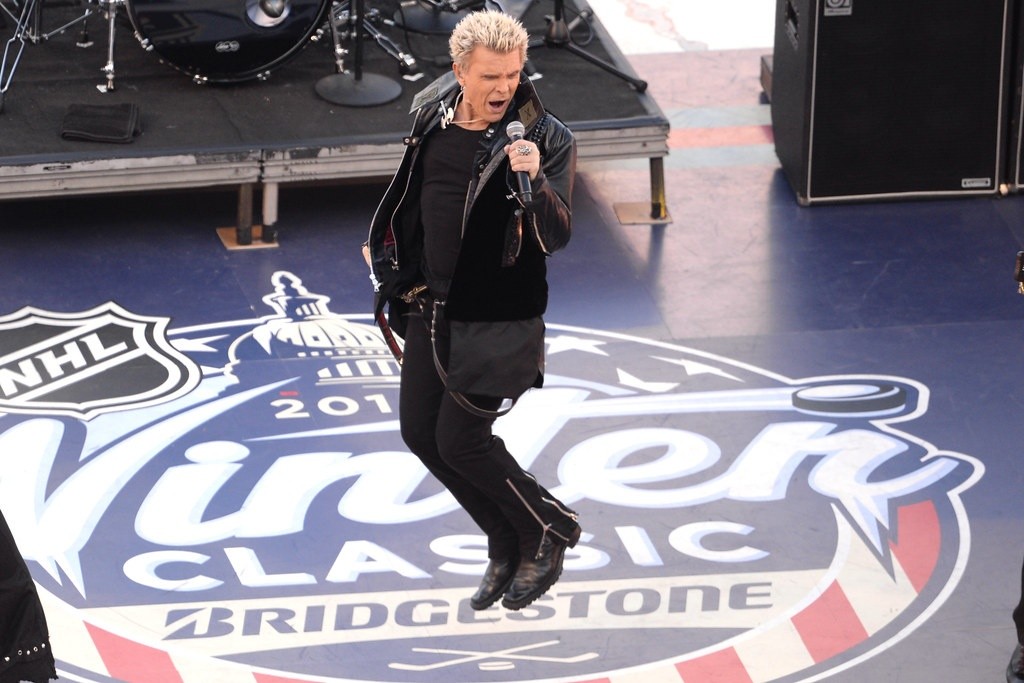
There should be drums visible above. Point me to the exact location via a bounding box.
[125,0,328,87]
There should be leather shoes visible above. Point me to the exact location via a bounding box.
[470,524,582,610]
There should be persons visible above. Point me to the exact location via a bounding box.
[362,8,583,610]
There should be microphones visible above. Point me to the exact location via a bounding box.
[506,121,532,204]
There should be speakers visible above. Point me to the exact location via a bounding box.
[771,0,1024,206]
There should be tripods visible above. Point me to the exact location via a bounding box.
[304,0,420,77]
[527,0,649,92]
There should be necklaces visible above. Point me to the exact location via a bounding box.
[440,91,483,129]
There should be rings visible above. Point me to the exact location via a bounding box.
[516,145,532,156]
